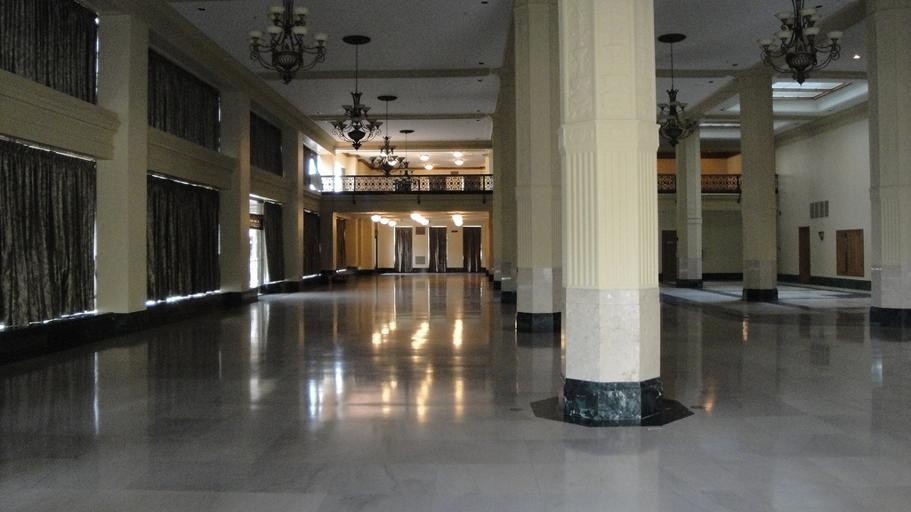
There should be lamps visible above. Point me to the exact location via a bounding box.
[245,0,385,153]
[658,1,845,149]
